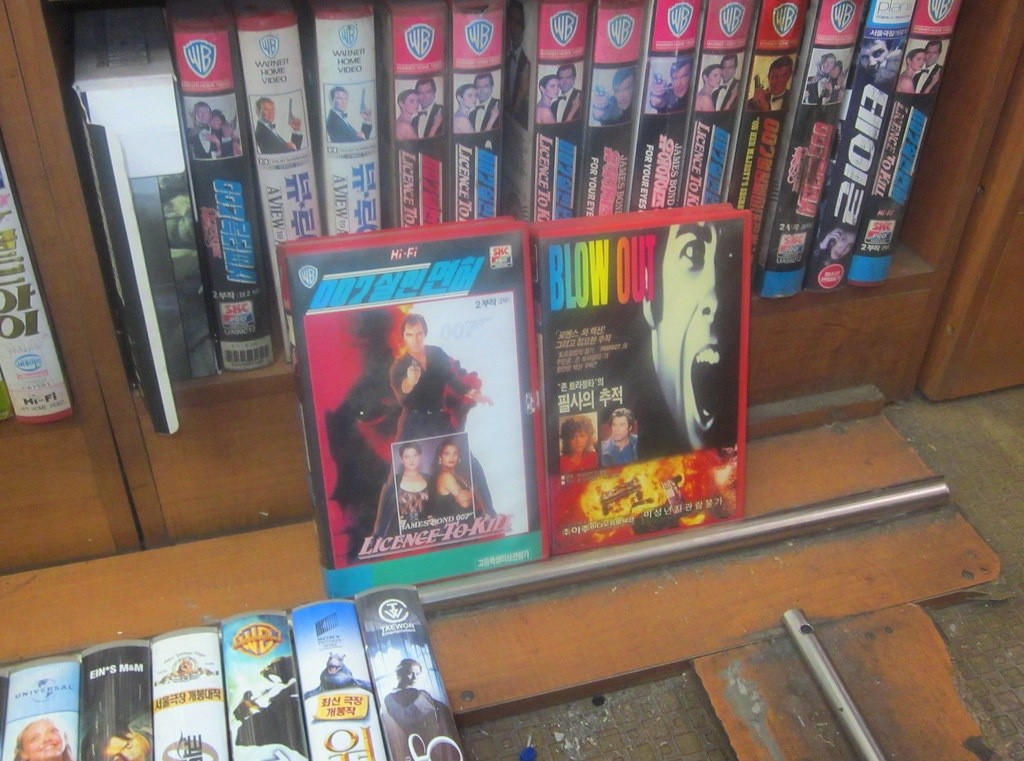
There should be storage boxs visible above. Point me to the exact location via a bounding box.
[70,6,224,436]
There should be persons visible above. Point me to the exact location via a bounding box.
[355,314,514,560]
[384,658,449,745]
[396,79,444,139]
[255,97,303,153]
[186,102,241,158]
[600,408,637,466]
[558,415,601,474]
[326,87,373,142]
[695,55,741,112]
[396,441,473,518]
[860,39,903,84]
[17,718,67,761]
[649,59,692,113]
[895,41,944,94]
[454,73,500,133]
[804,53,849,104]
[536,64,582,124]
[593,68,635,125]
[747,56,793,111]
[818,228,857,259]
